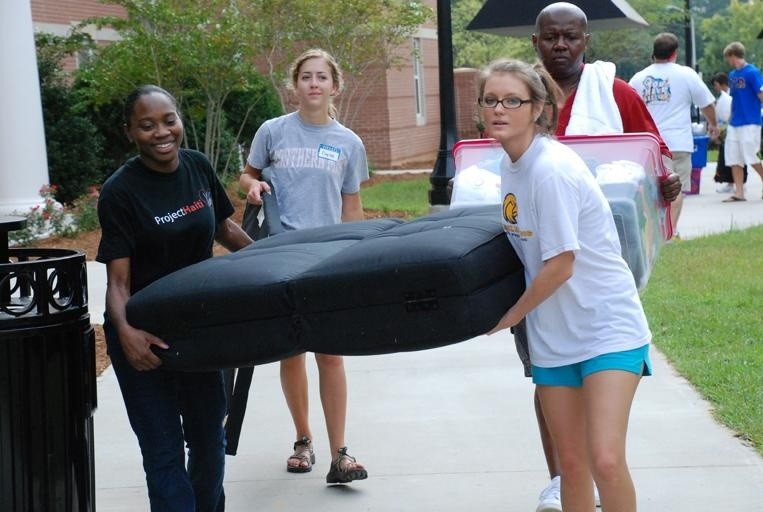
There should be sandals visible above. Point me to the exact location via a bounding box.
[325,448,368,484]
[286,436,315,473]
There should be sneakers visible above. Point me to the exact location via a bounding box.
[716,184,735,193]
[535,475,563,512]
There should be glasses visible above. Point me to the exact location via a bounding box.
[476,96,536,109]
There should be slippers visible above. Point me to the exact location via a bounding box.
[722,196,747,202]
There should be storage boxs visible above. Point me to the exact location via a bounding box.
[682,167,702,195]
[450,133,674,295]
[692,134,709,167]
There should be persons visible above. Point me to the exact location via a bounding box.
[710,71,747,194]
[628,32,721,245]
[445,2,681,512]
[95,83,254,511]
[472,57,654,512]
[239,48,368,482]
[721,41,763,202]
